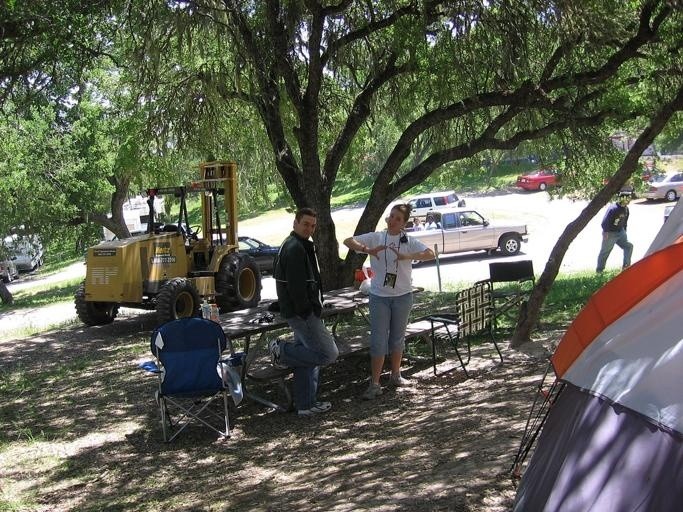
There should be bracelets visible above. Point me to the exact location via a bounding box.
[362,244,367,252]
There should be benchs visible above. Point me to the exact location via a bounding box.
[244,318,452,413]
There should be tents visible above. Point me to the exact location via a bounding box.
[508,190,683,512]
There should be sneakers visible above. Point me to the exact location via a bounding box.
[391,376,413,387]
[363,384,383,400]
[269,339,288,370]
[298,402,331,415]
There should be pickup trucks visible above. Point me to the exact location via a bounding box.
[403,206,532,270]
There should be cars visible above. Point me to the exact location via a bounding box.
[0,224,46,283]
[515,166,563,192]
[213,235,280,277]
[599,158,683,203]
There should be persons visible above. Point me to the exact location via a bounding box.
[413,215,424,233]
[341,202,434,400]
[270,206,338,416]
[425,215,437,229]
[597,190,633,273]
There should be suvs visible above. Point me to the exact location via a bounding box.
[382,190,467,223]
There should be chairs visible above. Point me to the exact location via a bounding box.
[141,316,247,443]
[470,259,534,330]
[411,281,506,379]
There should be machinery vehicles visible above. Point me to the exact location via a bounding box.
[70,157,263,325]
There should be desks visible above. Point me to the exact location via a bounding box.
[208,292,370,371]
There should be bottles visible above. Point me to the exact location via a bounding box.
[200,300,210,319]
[210,303,219,320]
